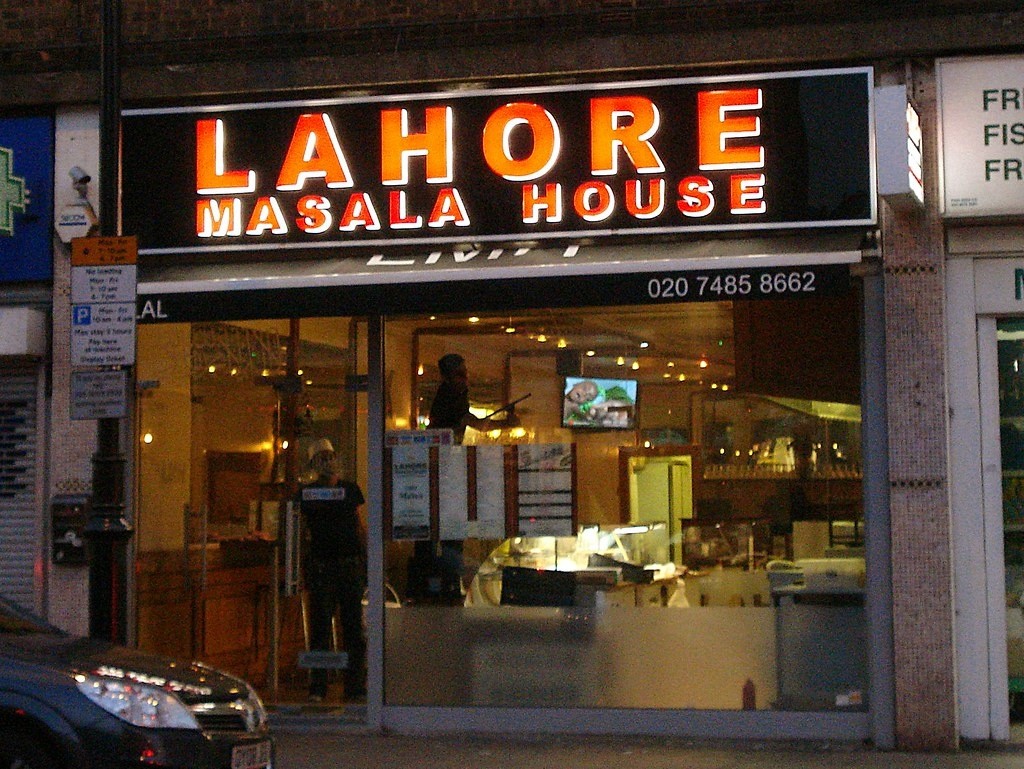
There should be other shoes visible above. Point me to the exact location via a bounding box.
[309,683,327,704]
[341,686,366,703]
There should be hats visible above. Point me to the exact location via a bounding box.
[306,438,334,464]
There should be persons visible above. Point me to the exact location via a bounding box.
[296,438,367,703]
[405,353,521,603]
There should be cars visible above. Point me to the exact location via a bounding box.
[0,595,279,769]
